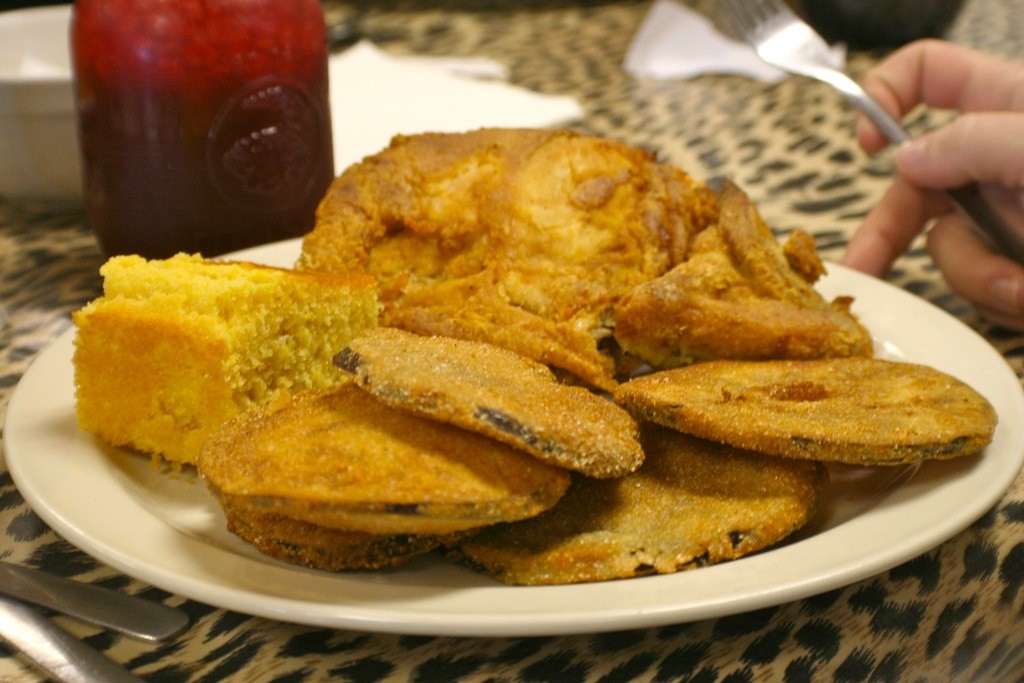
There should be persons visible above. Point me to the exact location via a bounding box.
[843,39,1024,331]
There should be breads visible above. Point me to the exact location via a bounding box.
[68,252,383,469]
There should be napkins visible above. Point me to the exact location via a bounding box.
[328,40,582,181]
